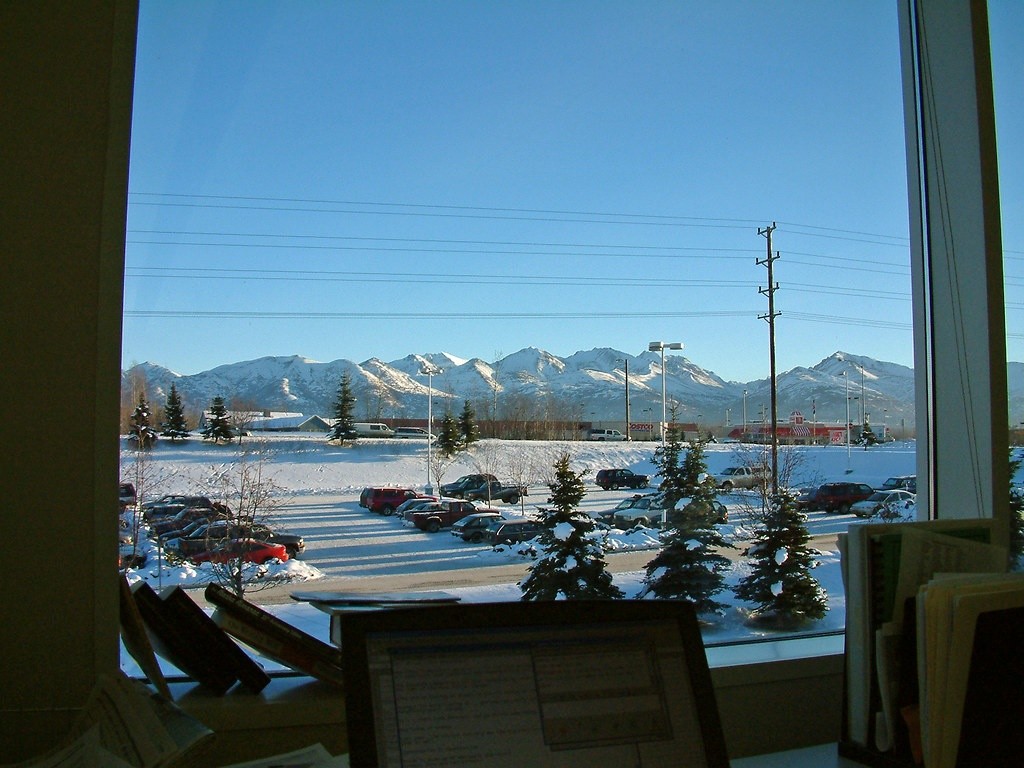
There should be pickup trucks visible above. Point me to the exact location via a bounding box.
[587,429,631,441]
[709,466,776,495]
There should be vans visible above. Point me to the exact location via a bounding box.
[354,423,392,438]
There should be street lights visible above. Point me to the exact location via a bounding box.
[757,404,769,440]
[883,409,888,443]
[643,407,655,440]
[616,357,630,442]
[420,369,443,495]
[725,407,731,428]
[743,389,747,434]
[648,342,684,448]
[840,362,868,459]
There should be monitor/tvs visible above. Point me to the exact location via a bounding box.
[337,598,732,768]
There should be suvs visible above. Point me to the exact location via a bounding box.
[593,468,651,490]
[393,427,437,439]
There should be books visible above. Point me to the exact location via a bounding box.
[838,519,1024,768]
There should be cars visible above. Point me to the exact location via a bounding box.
[607,494,677,531]
[119,484,147,571]
[142,493,304,566]
[357,473,611,548]
[793,476,919,519]
